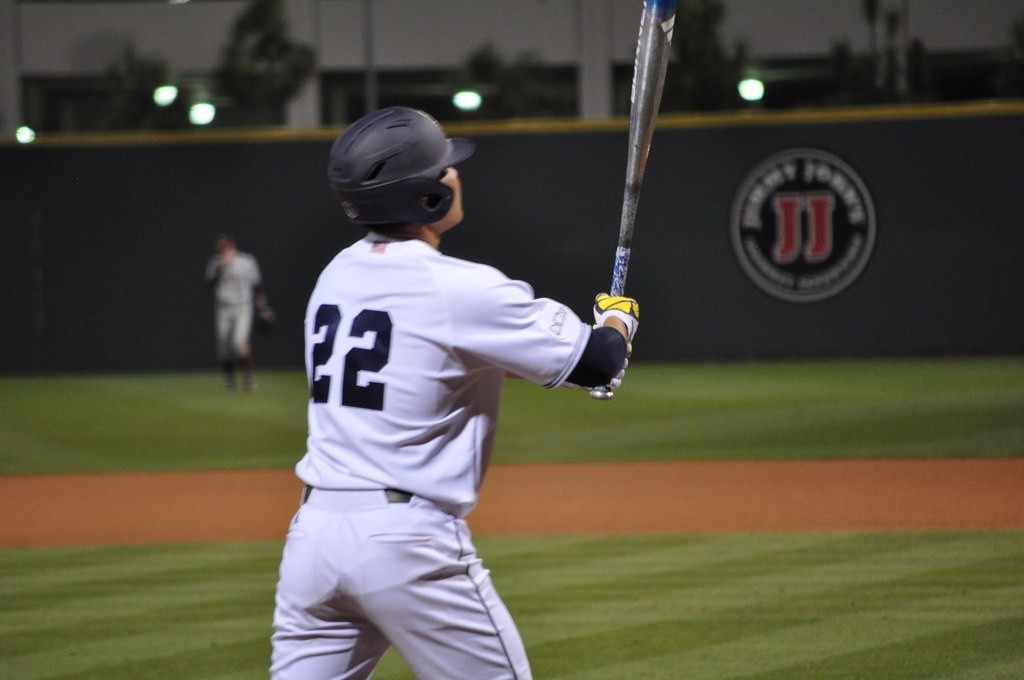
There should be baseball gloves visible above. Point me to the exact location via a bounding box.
[256,294,267,309]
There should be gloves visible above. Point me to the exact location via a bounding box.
[592,292,639,343]
[561,343,632,391]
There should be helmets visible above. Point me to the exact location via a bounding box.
[328,104,477,228]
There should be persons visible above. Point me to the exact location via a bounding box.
[269,108,638,680]
[204,235,279,389]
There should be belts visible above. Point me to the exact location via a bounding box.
[304,483,414,506]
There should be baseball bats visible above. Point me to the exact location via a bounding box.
[609,2,679,298]
